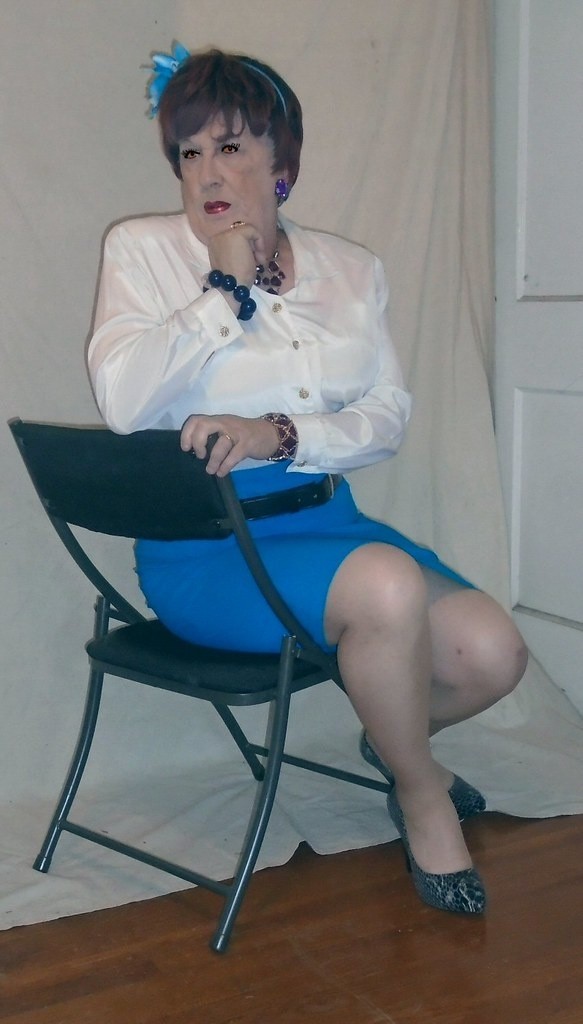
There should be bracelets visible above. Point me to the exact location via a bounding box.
[259,411,297,463]
[202,269,256,321]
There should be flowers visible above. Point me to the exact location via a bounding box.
[140,41,195,122]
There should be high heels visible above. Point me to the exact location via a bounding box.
[359,730,484,816]
[386,785,486,914]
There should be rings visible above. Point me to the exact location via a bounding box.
[231,220,245,229]
[226,434,231,440]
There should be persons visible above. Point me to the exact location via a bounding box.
[83,42,528,917]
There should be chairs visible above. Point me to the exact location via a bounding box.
[6,399,343,956]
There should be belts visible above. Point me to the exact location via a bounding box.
[240,473,343,520]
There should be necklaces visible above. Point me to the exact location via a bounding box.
[255,238,285,295]
